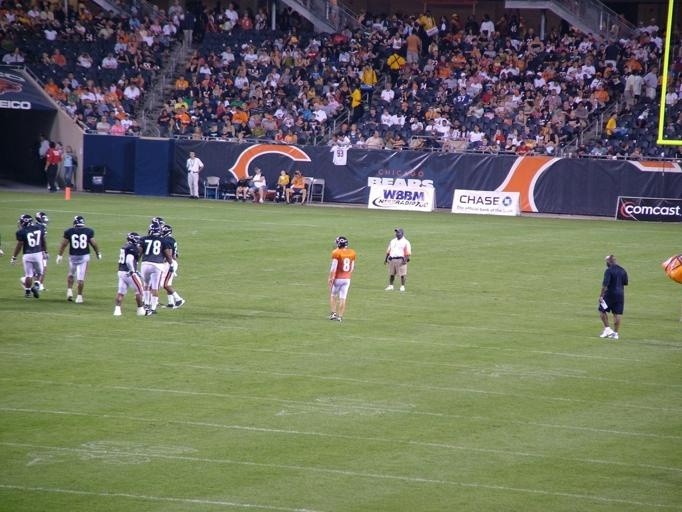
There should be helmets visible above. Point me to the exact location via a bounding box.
[161,225,173,238]
[334,235,349,248]
[34,212,49,227]
[17,214,34,230]
[148,217,165,237]
[127,232,141,245]
[72,215,85,227]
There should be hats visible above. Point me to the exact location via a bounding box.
[394,227,404,236]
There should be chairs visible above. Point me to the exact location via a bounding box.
[203,176,220,199]
[223,176,325,205]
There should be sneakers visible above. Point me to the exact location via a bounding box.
[329,312,343,322]
[24,290,32,298]
[399,284,406,293]
[31,283,41,298]
[161,302,174,309]
[383,284,394,292]
[600,327,621,342]
[172,299,185,310]
[75,295,84,304]
[112,305,122,316]
[137,301,157,316]
[66,288,73,302]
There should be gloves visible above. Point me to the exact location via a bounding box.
[384,253,390,265]
[403,256,410,265]
[56,254,63,265]
[95,251,102,260]
[169,264,176,273]
[10,256,18,265]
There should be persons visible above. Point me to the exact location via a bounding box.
[57,216,101,304]
[10,215,47,299]
[21,212,49,290]
[384,229,411,292]
[0,0,682,204]
[598,255,628,340]
[112,218,186,316]
[328,237,355,322]
